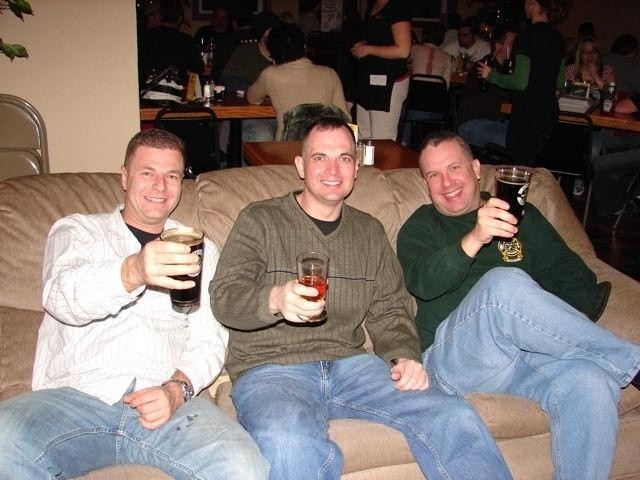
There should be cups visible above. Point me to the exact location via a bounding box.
[501,58,515,78]
[296,250,330,323]
[194,33,227,104]
[457,51,471,77]
[478,58,492,91]
[159,225,206,315]
[494,167,532,238]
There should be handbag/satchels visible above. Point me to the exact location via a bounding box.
[141,70,189,107]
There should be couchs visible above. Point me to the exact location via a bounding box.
[0,164,639,480]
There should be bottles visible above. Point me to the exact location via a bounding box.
[598,81,618,115]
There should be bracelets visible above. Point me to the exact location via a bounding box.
[169,380,194,401]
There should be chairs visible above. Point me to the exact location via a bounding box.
[395,73,451,151]
[1,95,49,178]
[444,84,508,192]
[547,110,594,229]
[599,136,638,233]
[282,103,353,141]
[150,104,219,177]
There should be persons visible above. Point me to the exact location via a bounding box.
[395,130,639,479]
[0,129,271,479]
[209,116,513,479]
[134,2,640,159]
